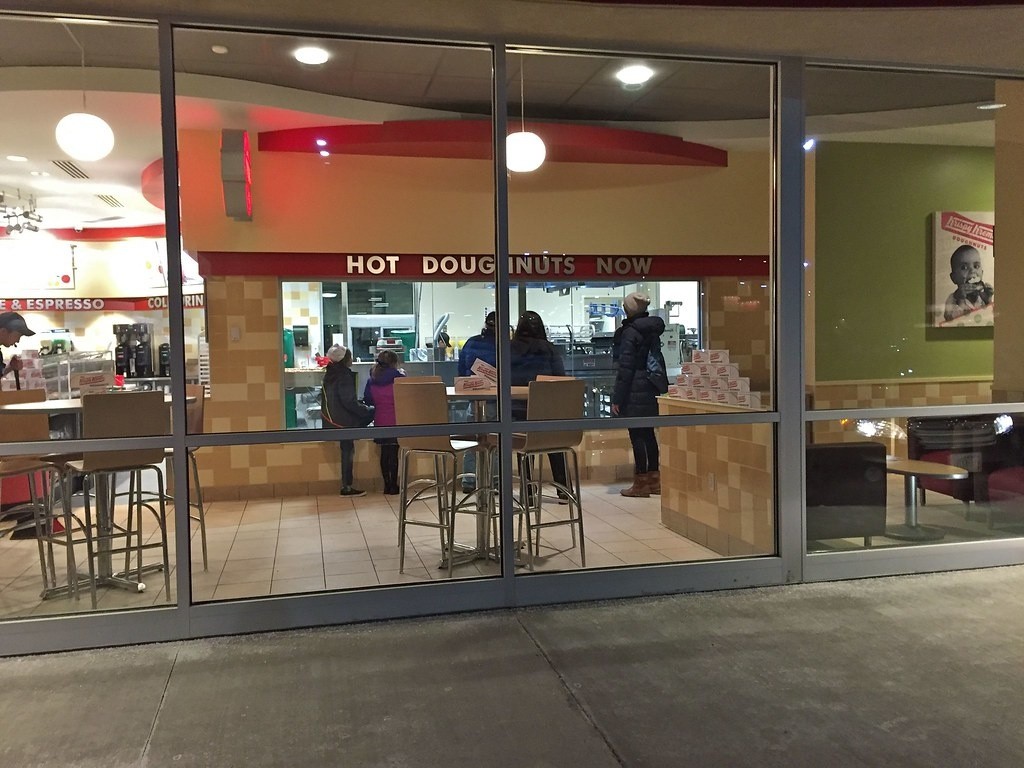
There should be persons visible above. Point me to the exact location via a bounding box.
[509,309,578,504]
[942,244,994,322]
[436,332,454,358]
[610,293,670,499]
[364,351,408,495]
[319,343,366,498]
[458,312,514,495]
[0,311,24,393]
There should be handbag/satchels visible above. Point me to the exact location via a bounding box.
[647,348,670,394]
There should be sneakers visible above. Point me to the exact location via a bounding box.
[340,487,366,497]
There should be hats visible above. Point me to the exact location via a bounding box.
[438,332,452,347]
[327,343,351,363]
[0,312,36,337]
[624,292,650,315]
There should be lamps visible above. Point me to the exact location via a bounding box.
[54,52,116,160]
[506,55,550,174]
[3,199,50,237]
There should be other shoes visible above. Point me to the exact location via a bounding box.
[522,495,537,506]
[462,484,500,495]
[383,486,400,495]
[559,493,577,504]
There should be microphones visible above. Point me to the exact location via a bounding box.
[14,344,17,347]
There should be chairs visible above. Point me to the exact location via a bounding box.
[0,388,66,592]
[58,387,172,609]
[485,372,579,557]
[0,446,89,602]
[904,412,1019,523]
[482,377,589,571]
[110,381,215,582]
[806,438,888,546]
[970,417,1024,533]
[390,381,492,576]
[392,374,478,549]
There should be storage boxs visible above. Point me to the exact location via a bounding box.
[79,372,119,406]
[469,358,496,382]
[668,348,761,408]
[452,376,490,391]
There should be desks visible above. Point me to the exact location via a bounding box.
[434,384,542,570]
[0,397,167,600]
[0,395,198,599]
[886,456,969,542]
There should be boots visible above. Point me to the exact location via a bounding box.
[619,471,662,498]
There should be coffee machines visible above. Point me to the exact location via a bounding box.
[113,323,155,378]
